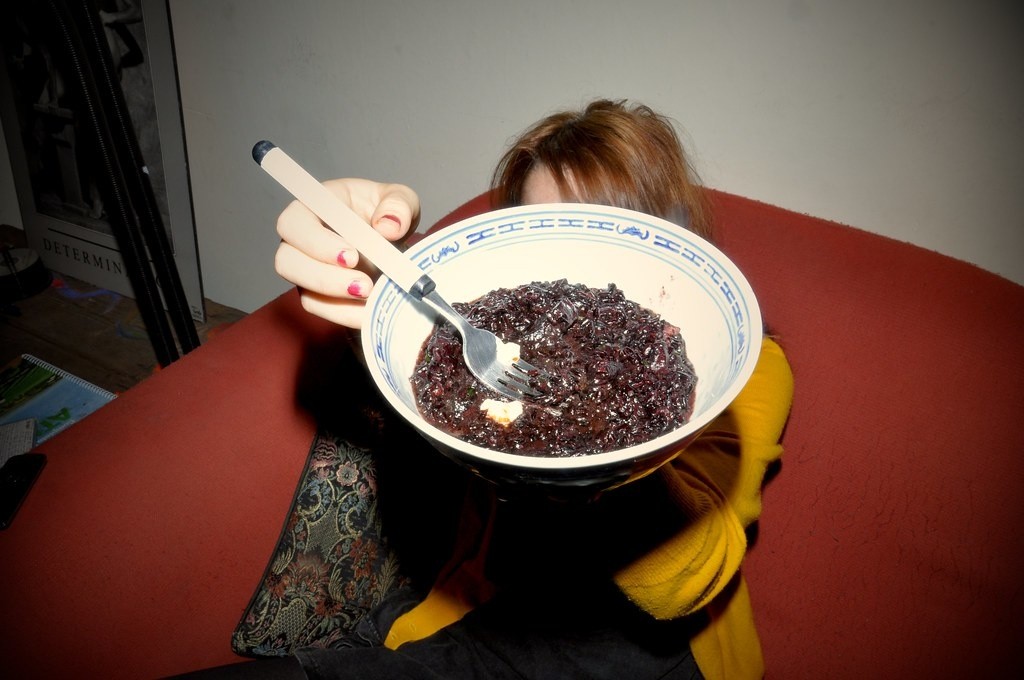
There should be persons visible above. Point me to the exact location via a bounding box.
[10,1,142,219]
[167,96,795,680]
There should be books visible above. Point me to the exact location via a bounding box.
[0,353,118,448]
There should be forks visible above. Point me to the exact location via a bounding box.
[250,138,555,401]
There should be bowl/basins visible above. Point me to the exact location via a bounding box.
[360,208,764,495]
